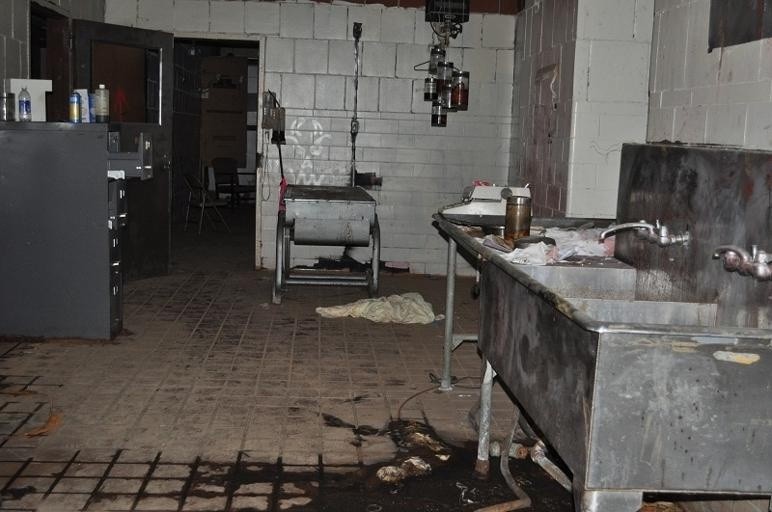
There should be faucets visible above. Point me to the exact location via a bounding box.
[598,222,655,242]
[712,245,751,266]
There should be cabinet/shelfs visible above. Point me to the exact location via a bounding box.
[105,128,154,343]
[200,54,247,167]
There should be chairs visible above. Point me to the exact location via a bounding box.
[173,155,241,235]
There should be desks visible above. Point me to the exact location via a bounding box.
[215,185,257,215]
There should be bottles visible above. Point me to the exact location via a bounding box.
[0,92,14,122]
[505,195,530,240]
[18,86,32,122]
[69,93,80,124]
[422,44,470,128]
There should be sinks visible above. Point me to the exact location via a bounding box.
[563,295,766,337]
[510,264,717,303]
